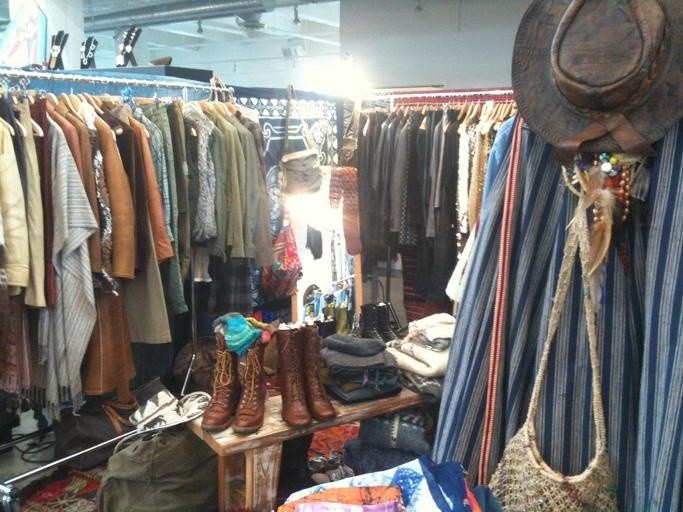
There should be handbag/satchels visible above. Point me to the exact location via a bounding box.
[488,422,617,511]
[56,375,168,470]
[343,137,357,166]
[282,150,319,195]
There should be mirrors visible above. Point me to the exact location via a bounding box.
[276,163,363,338]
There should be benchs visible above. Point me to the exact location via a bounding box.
[176,360,438,510]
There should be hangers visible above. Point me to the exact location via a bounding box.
[0,68,234,139]
[359,90,520,138]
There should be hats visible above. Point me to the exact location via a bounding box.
[510,0,681,155]
[113,388,212,453]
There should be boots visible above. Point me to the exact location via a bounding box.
[279,433,317,491]
[360,302,398,344]
[277,324,336,427]
[199,323,241,430]
[232,336,266,433]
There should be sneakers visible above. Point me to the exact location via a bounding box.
[308,451,355,481]
